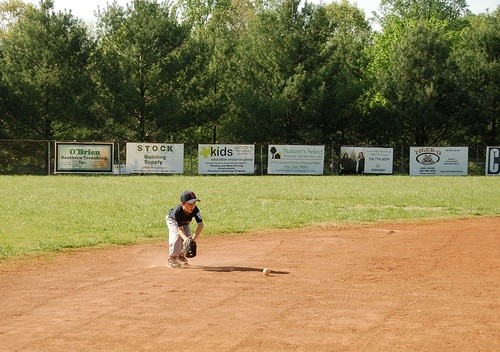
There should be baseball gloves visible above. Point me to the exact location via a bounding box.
[182,236,197,258]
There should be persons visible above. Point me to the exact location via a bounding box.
[340,152,357,173]
[165,189,203,269]
[358,152,365,173]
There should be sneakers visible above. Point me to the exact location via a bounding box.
[168,256,181,269]
[177,256,188,265]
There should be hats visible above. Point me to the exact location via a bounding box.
[180,191,201,204]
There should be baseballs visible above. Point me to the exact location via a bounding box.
[263,268,271,275]
